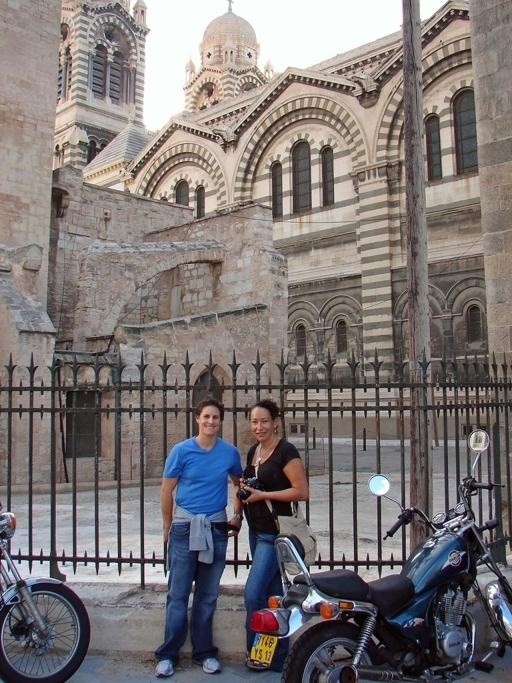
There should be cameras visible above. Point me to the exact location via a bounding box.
[235,478,263,502]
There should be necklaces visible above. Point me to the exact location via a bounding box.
[257,435,276,462]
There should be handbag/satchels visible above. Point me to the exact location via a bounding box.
[276,501,317,575]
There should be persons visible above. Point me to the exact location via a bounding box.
[153,401,245,678]
[237,400,310,673]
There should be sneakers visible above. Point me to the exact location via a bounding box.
[193,656,221,674]
[155,660,175,678]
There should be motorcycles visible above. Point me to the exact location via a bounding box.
[0,511,91,683]
[250,430,512,682]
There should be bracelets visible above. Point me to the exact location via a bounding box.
[233,512,243,521]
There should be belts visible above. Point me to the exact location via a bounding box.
[211,523,240,532]
